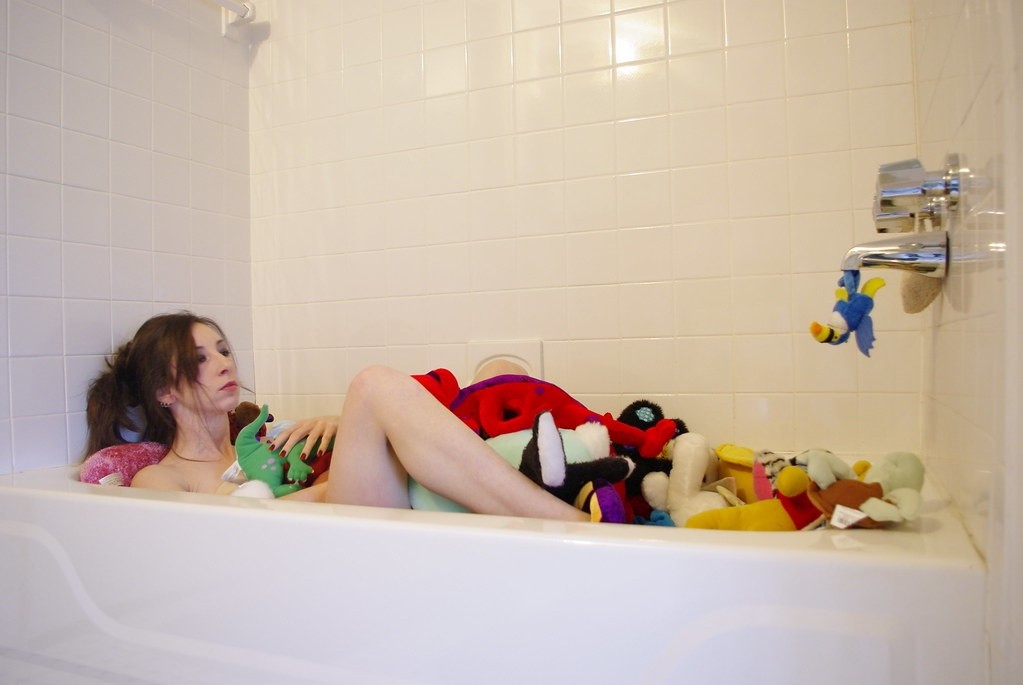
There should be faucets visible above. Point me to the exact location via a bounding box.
[839,229,950,278]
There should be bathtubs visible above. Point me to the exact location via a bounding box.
[0,413,994,685]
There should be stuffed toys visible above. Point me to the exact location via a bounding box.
[810,268,885,361]
[230,400,334,494]
[414,365,932,538]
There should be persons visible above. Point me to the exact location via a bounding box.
[83,310,594,522]
